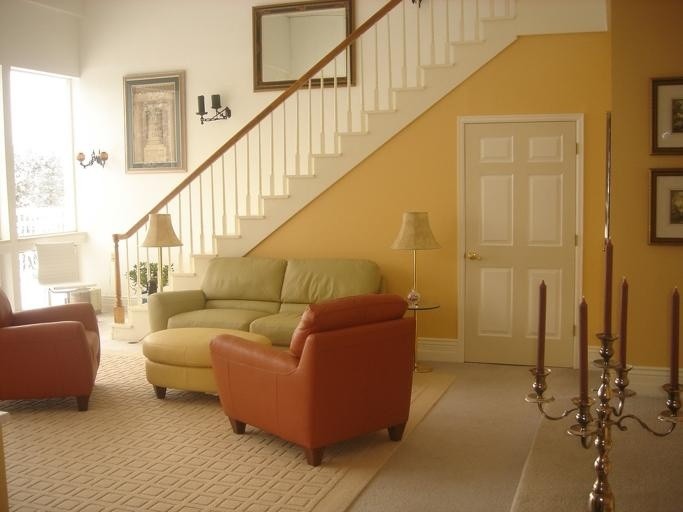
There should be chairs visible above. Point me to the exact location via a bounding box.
[37,242,101,316]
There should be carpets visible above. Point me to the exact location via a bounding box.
[1,347,462,512]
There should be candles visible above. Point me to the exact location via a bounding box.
[578,297,589,400]
[536,280,546,373]
[620,274,629,369]
[670,282,679,388]
[604,231,613,335]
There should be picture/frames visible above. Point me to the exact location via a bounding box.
[252,1,358,92]
[650,166,683,245]
[124,70,189,175]
[651,79,681,157]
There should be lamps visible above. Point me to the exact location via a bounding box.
[390,211,442,372]
[77,149,109,169]
[141,212,184,293]
[196,94,230,124]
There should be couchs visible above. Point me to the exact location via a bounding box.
[0,290,101,410]
[141,255,383,399]
[209,293,416,464]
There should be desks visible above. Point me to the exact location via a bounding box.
[509,395,683,510]
